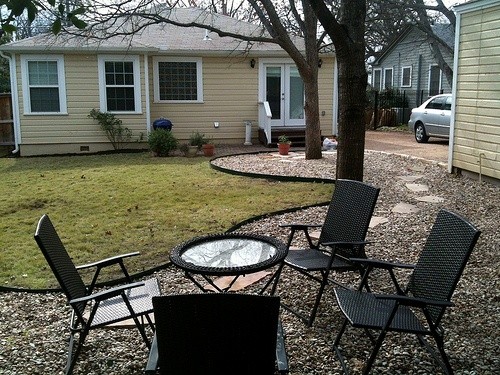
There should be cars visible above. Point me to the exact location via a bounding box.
[408,93,452,143]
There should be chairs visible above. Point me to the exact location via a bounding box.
[269,179,380,325]
[144,293,290,375]
[34,213,162,375]
[327,208,481,375]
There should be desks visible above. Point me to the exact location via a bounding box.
[170,232,287,293]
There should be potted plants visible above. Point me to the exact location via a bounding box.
[184,131,215,157]
[277,135,292,155]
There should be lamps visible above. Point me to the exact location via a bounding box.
[250,59,255,68]
[318,59,322,67]
